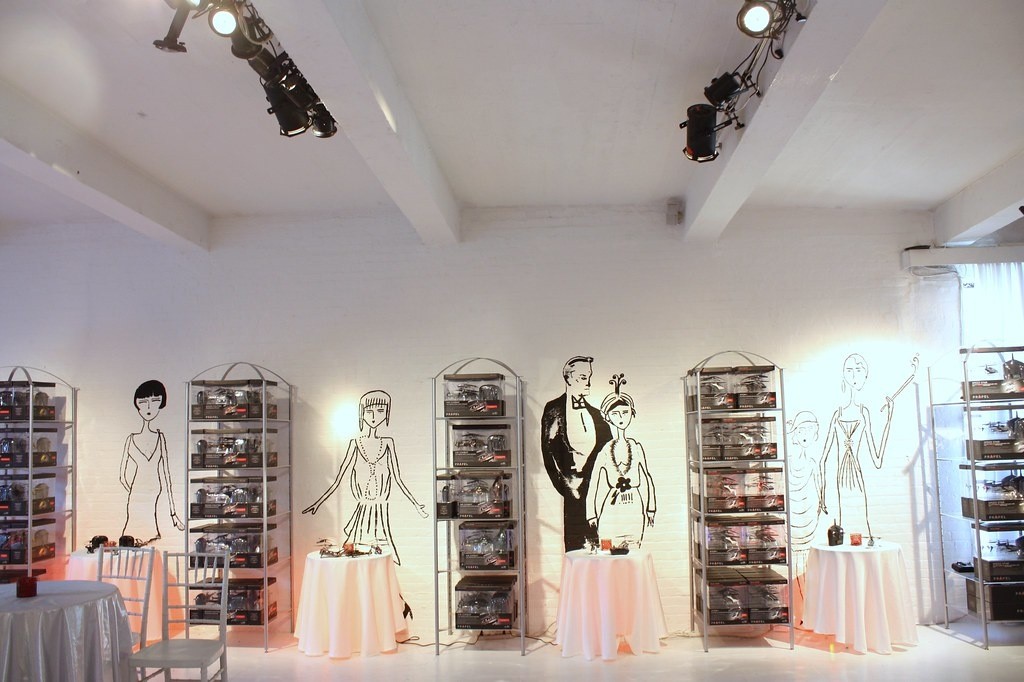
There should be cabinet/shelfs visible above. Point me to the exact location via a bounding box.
[0,364,76,583]
[928,331,1024,651]
[431,358,530,656]
[184,362,295,653]
[682,348,796,654]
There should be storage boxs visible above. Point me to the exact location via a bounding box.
[442,374,519,630]
[686,365,788,625]
[955,346,1024,621]
[190,380,280,626]
[0,381,57,583]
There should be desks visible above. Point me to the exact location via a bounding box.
[67,551,184,648]
[294,550,408,659]
[799,543,918,655]
[560,549,666,662]
[0,580,141,682]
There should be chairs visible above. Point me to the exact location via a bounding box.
[97,544,231,682]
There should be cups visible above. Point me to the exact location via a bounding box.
[17,577,36,597]
[103,541,116,552]
[601,540,612,551]
[343,543,354,552]
[849,533,862,546]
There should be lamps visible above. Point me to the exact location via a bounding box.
[679,0,807,162]
[154,1,337,139]
